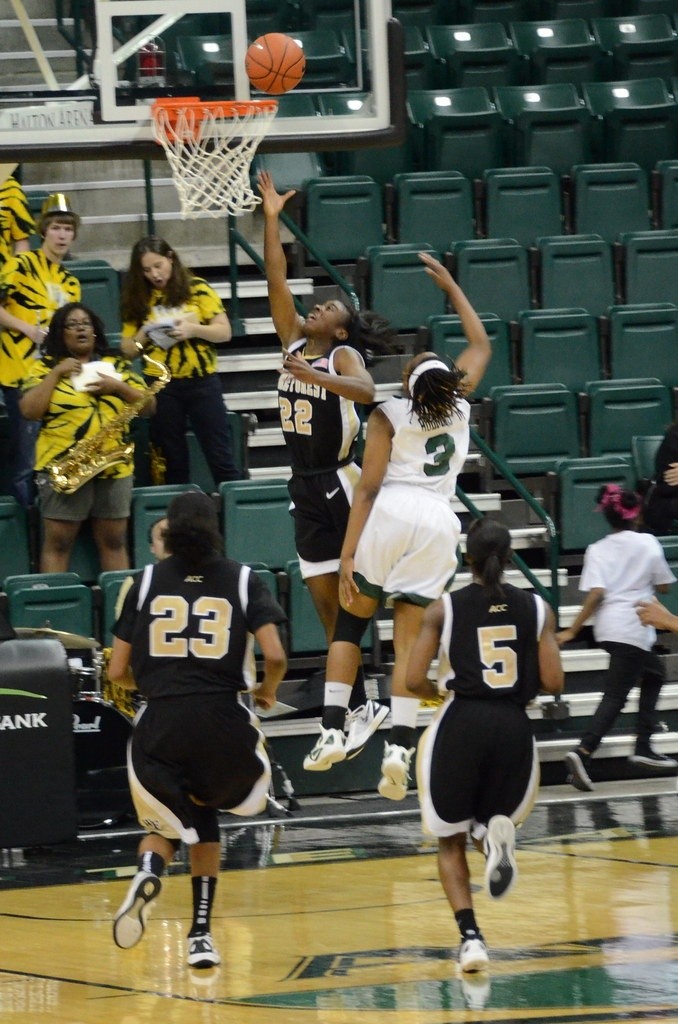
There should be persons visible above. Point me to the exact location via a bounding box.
[641,420,678,533]
[302,249,492,801]
[406,522,566,970]
[632,595,678,633]
[256,168,397,758]
[0,172,287,965]
[555,484,676,790]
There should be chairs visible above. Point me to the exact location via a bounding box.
[0,0,678,672]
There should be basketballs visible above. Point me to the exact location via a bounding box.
[244,31,308,95]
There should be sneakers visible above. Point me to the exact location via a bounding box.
[186,932,220,967]
[302,725,347,773]
[565,749,594,792]
[631,743,678,768]
[189,968,221,991]
[345,701,389,760]
[458,935,489,974]
[484,815,518,901]
[378,738,415,801]
[112,872,163,951]
[455,962,492,1011]
